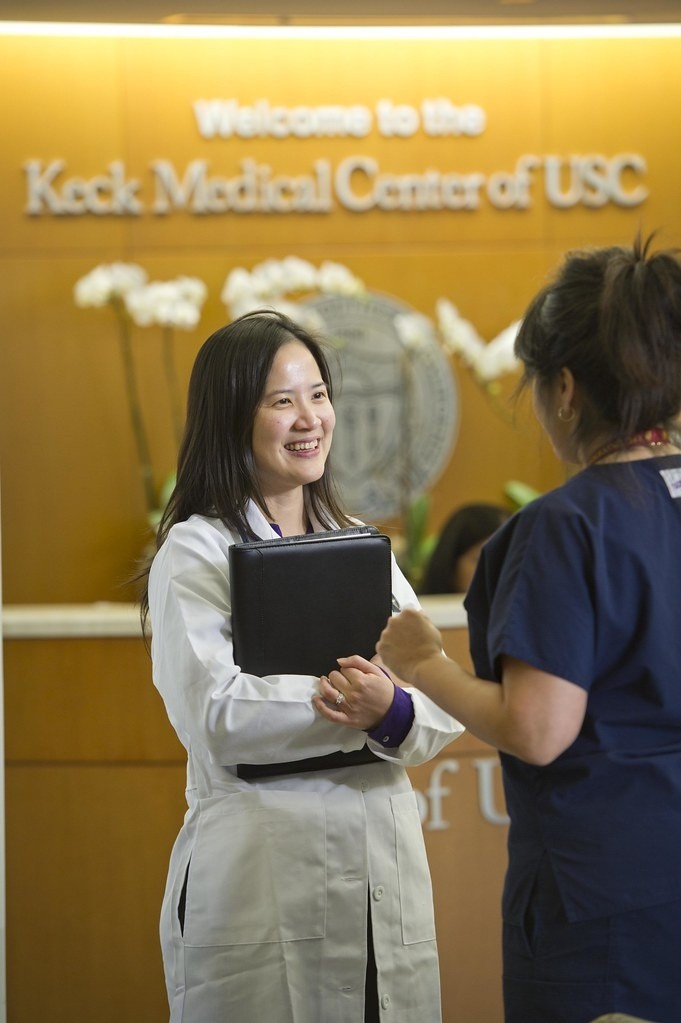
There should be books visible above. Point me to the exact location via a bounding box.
[227,525,392,778]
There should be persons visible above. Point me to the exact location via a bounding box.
[415,501,514,596]
[374,222,681,1023]
[122,309,468,1023]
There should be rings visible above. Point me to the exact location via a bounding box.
[335,692,344,706]
